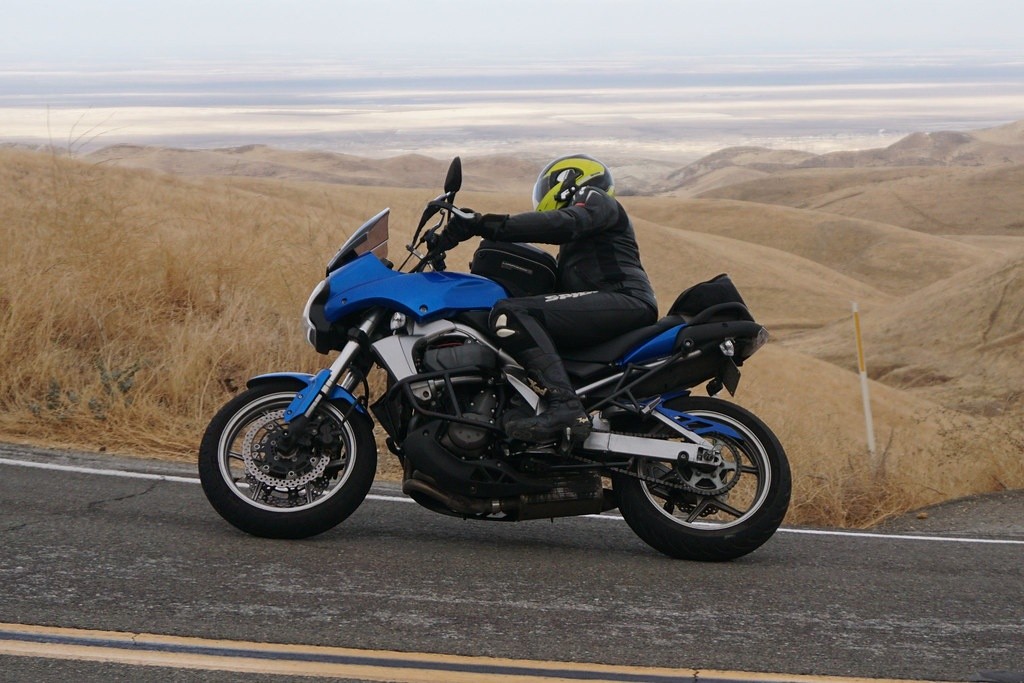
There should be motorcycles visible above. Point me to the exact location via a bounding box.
[194,153,792,562]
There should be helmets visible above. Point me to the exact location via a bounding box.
[531,152,613,212]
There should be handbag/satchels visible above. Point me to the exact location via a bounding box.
[469,240,563,298]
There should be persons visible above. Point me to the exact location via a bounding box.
[441,156,659,441]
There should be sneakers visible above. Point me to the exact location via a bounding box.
[506,397,590,440]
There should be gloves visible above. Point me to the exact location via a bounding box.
[445,208,482,242]
